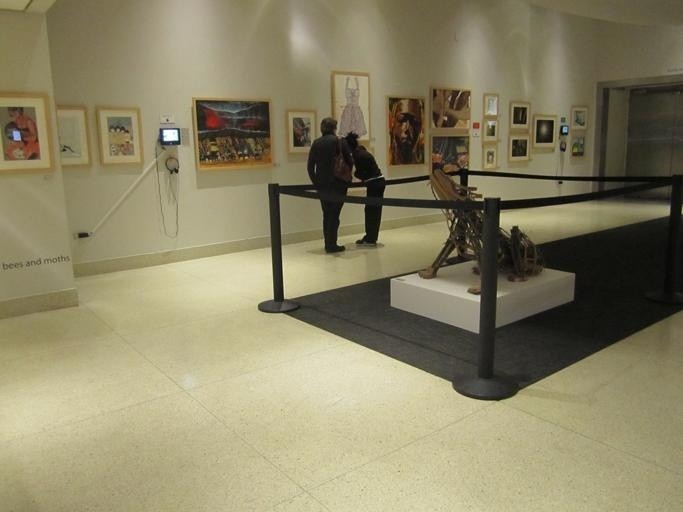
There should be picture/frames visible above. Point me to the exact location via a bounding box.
[427,133,472,177]
[188,93,279,172]
[0,91,56,174]
[329,69,372,148]
[384,94,427,169]
[54,103,94,169]
[478,85,592,171]
[283,108,320,157]
[428,85,473,133]
[93,105,144,168]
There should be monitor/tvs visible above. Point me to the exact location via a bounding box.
[159,128,182,145]
[560,125,569,135]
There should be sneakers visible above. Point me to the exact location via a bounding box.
[356,237,376,246]
[325,244,344,252]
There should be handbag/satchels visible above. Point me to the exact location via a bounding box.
[333,153,351,182]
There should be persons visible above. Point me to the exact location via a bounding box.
[5,107,40,160]
[305,116,354,254]
[108,124,131,156]
[390,99,423,164]
[342,130,386,248]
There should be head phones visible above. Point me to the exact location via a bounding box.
[166,157,179,174]
[560,142,566,152]
